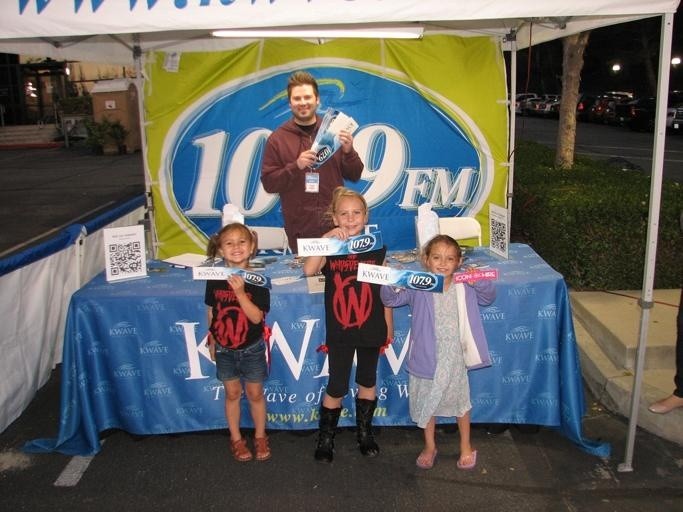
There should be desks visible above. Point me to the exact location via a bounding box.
[21,239,611,463]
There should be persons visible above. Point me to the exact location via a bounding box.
[304,185,393,463]
[647,284,681,415]
[201,223,273,462]
[378,235,496,468]
[260,73,363,255]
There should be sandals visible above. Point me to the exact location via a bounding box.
[229,436,253,463]
[252,434,273,462]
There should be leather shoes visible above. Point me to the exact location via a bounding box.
[648,393,683,414]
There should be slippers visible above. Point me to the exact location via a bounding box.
[455,448,479,470]
[414,447,439,470]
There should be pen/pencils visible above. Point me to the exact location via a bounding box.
[146,268,167,272]
[169,264,187,269]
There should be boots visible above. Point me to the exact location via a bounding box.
[313,400,345,466]
[354,393,381,459]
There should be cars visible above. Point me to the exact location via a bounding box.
[508,89,683,129]
